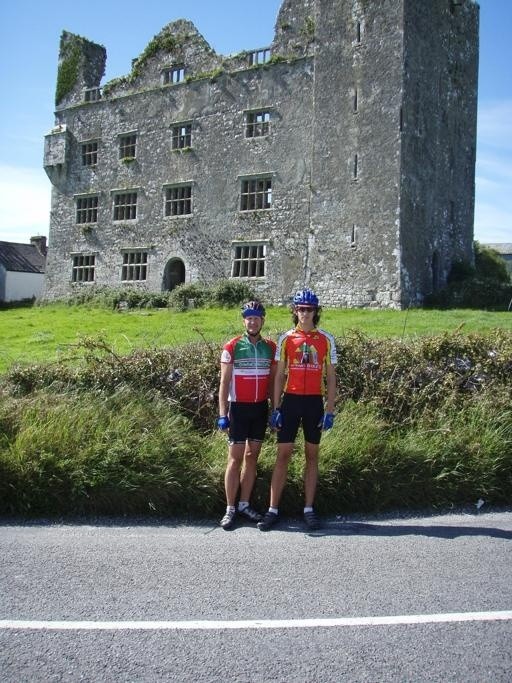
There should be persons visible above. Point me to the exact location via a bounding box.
[217,301,280,529]
[254,290,339,530]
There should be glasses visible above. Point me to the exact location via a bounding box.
[297,307,315,312]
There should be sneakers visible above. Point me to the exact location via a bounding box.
[257,510,280,531]
[303,511,321,529]
[220,510,236,529]
[236,506,263,523]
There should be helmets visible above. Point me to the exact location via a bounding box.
[293,290,317,304]
[241,301,265,317]
